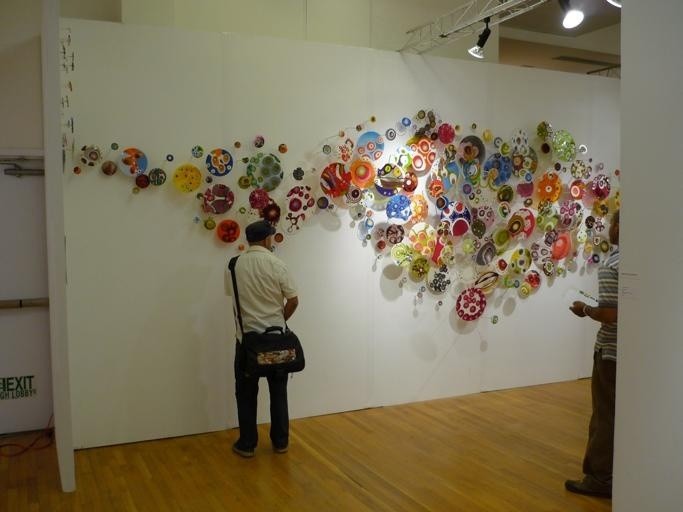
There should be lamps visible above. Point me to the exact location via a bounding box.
[466,18,491,61]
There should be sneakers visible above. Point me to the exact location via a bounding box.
[272,440,289,453]
[233,440,255,457]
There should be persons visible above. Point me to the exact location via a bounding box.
[564,209,618,499]
[221,218,299,459]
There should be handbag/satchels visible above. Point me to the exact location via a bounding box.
[239,326,305,379]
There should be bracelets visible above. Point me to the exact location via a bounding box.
[582,304,591,315]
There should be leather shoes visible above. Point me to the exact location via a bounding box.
[564,479,611,498]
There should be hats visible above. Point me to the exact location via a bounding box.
[246,221,276,242]
